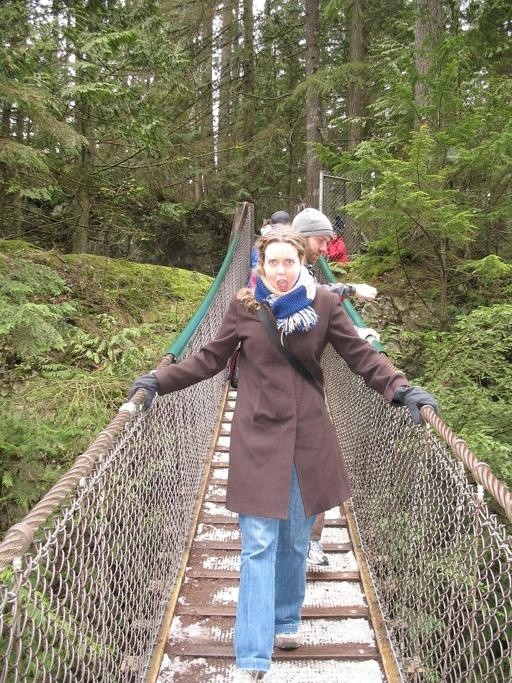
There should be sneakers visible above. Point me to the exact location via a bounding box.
[231,670,264,683]
[306,541,328,565]
[274,633,301,650]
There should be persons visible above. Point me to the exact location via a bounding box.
[121,226,439,683]
[244,208,380,565]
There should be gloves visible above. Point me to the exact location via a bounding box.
[394,385,438,425]
[127,374,160,408]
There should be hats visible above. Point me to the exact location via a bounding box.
[292,208,334,241]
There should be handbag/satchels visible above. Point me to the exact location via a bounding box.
[227,344,242,389]
